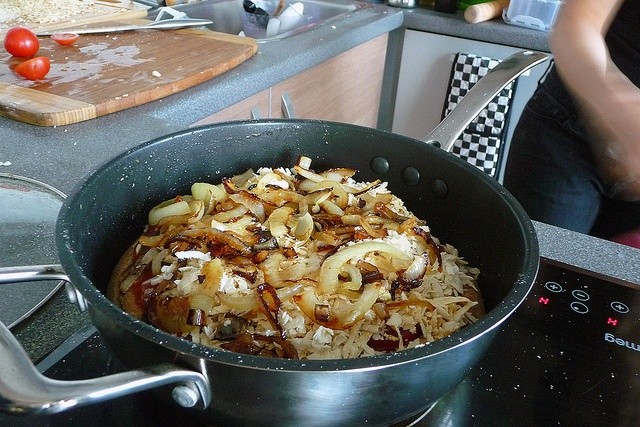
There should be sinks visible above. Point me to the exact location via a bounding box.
[139,0,364,43]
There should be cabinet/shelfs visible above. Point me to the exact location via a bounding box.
[188,31,390,130]
[391,29,555,185]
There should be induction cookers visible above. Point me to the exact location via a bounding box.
[1,256,639,427]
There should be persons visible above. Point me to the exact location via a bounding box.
[502,0,639,235]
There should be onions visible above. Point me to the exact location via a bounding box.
[107,155,486,361]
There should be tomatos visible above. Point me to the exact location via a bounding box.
[15,56,50,80]
[51,32,80,46]
[3,26,39,57]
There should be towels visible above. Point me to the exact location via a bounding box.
[444,52,516,180]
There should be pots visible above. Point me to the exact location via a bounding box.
[1,50,549,426]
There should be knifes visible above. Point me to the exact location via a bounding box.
[34,18,214,36]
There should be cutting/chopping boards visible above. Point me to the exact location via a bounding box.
[0,16,258,127]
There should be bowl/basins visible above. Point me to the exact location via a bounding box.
[240,3,321,39]
